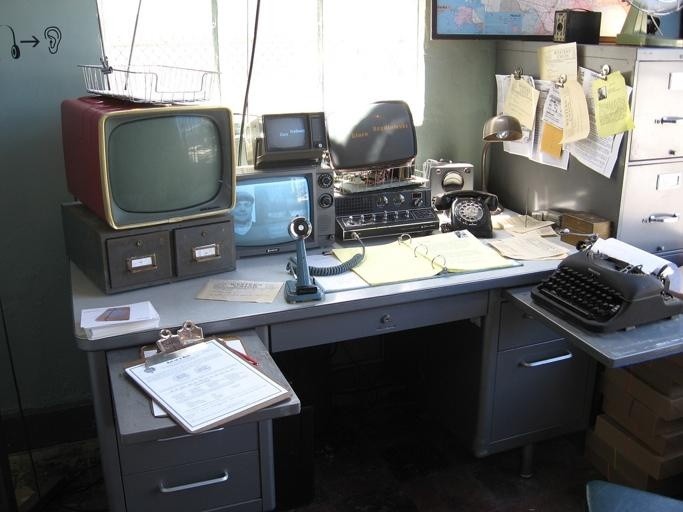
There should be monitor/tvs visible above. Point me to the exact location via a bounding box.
[61,95,236,231]
[325,98,425,195]
[234,163,336,259]
[243,112,326,167]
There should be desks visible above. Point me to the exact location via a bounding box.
[67,202,599,510]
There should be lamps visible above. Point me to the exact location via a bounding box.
[480,109,524,211]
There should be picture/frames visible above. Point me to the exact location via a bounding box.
[429,0,625,42]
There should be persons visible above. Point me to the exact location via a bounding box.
[231,192,267,242]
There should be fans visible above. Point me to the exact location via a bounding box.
[618,0,683,48]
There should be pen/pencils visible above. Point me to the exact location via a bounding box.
[230,348,257,365]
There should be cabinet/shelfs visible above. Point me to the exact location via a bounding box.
[490,36,683,264]
[60,198,236,297]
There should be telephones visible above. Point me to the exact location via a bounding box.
[434,190,498,238]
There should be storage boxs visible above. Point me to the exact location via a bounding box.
[602,356,682,456]
[588,410,681,494]
[559,210,610,250]
[617,338,683,398]
